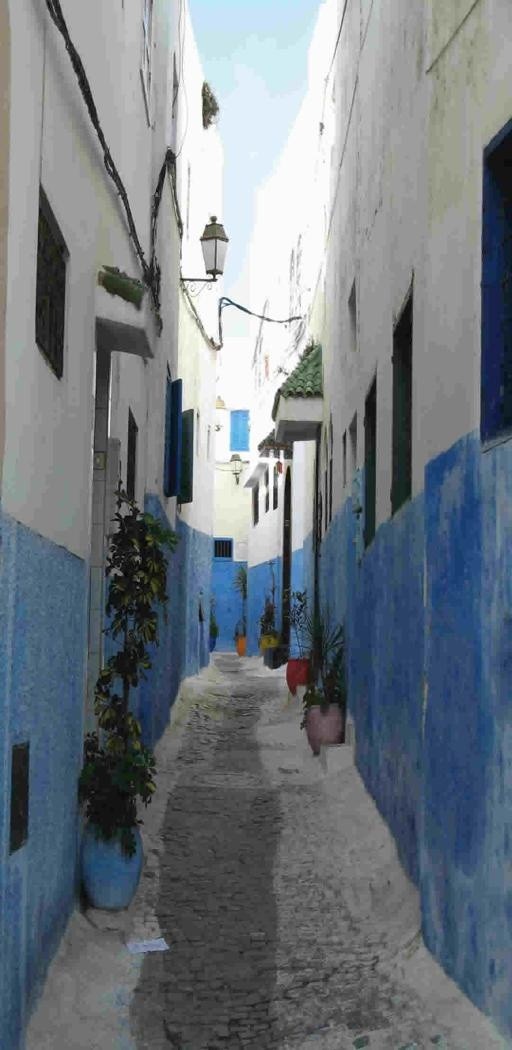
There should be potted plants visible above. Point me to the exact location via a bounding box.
[280,587,309,695]
[288,597,348,755]
[210,592,218,652]
[231,565,247,657]
[255,595,282,668]
[76,477,182,910]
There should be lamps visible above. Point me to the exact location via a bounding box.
[230,454,242,485]
[181,216,229,297]
